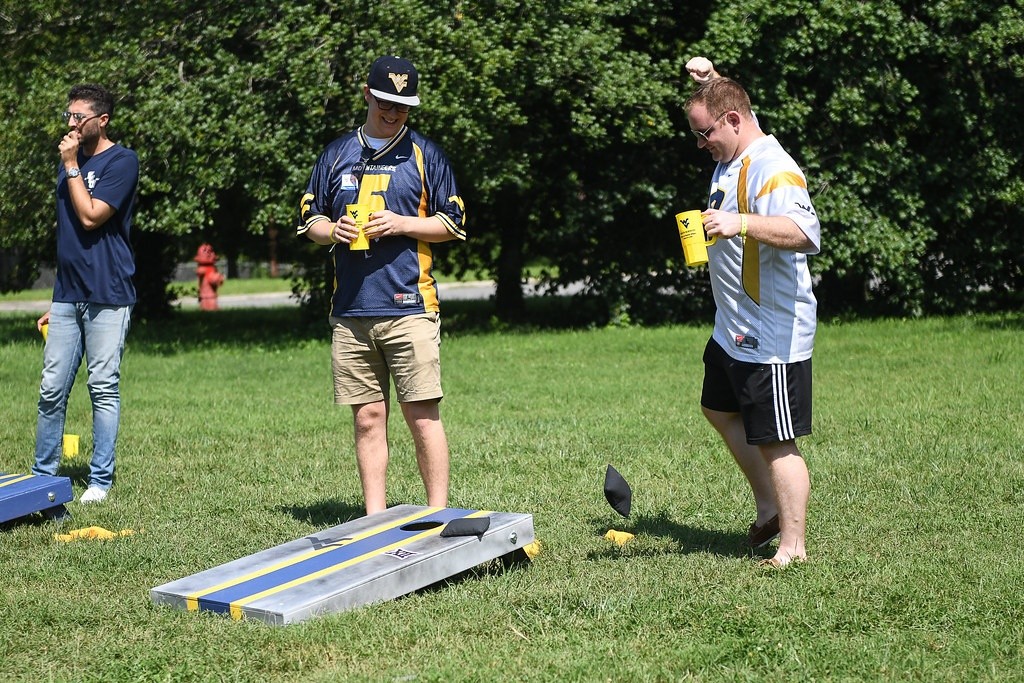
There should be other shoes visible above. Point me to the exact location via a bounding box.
[741,513,782,550]
[757,559,784,572]
[80,487,107,504]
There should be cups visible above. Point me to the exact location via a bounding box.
[674,209,717,266]
[62,434,79,458]
[603,529,634,545]
[40,324,48,343]
[345,204,376,251]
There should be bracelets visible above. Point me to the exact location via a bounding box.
[329,224,340,244]
[738,214,747,238]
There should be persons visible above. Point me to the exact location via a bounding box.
[682,56,821,571]
[295,55,468,517]
[30,82,140,509]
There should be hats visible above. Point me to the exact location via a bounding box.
[367,55,420,106]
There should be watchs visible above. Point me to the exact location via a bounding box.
[65,167,82,179]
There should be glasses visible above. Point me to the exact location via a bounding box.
[372,94,412,113]
[61,111,100,119]
[690,112,728,143]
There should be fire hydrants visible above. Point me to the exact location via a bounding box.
[194,241,224,308]
